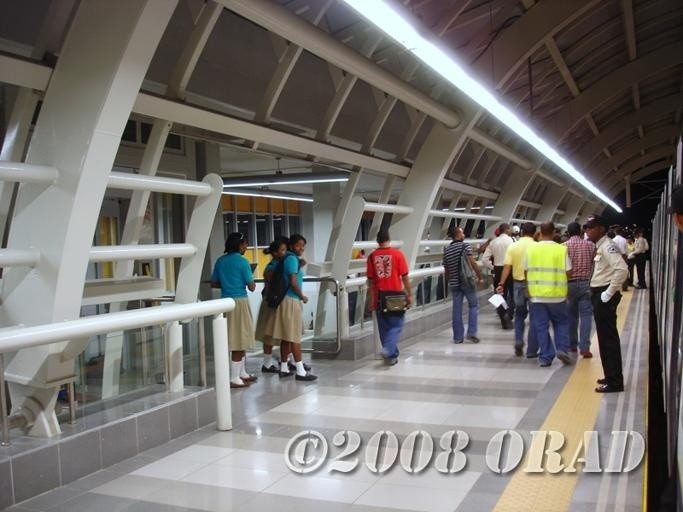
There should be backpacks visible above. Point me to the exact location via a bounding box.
[267,253,300,309]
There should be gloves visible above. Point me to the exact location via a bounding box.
[600,291,611,303]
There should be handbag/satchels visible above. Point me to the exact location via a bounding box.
[377,290,409,315]
[458,243,476,289]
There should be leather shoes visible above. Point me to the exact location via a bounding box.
[596,385,623,392]
[503,313,513,329]
[466,336,479,343]
[597,379,608,385]
[240,374,257,382]
[261,362,317,381]
[230,379,251,388]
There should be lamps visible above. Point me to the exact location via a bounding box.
[222,187,313,202]
[223,156,351,187]
[345,0,624,213]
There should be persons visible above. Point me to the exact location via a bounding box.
[210,217,649,392]
[668,182,683,234]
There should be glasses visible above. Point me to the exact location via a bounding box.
[583,224,594,230]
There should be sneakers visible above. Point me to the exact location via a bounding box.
[514,341,592,367]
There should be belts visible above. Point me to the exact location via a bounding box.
[568,279,590,283]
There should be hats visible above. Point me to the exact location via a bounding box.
[582,215,609,227]
[511,226,520,234]
[665,189,682,215]
[565,222,579,233]
